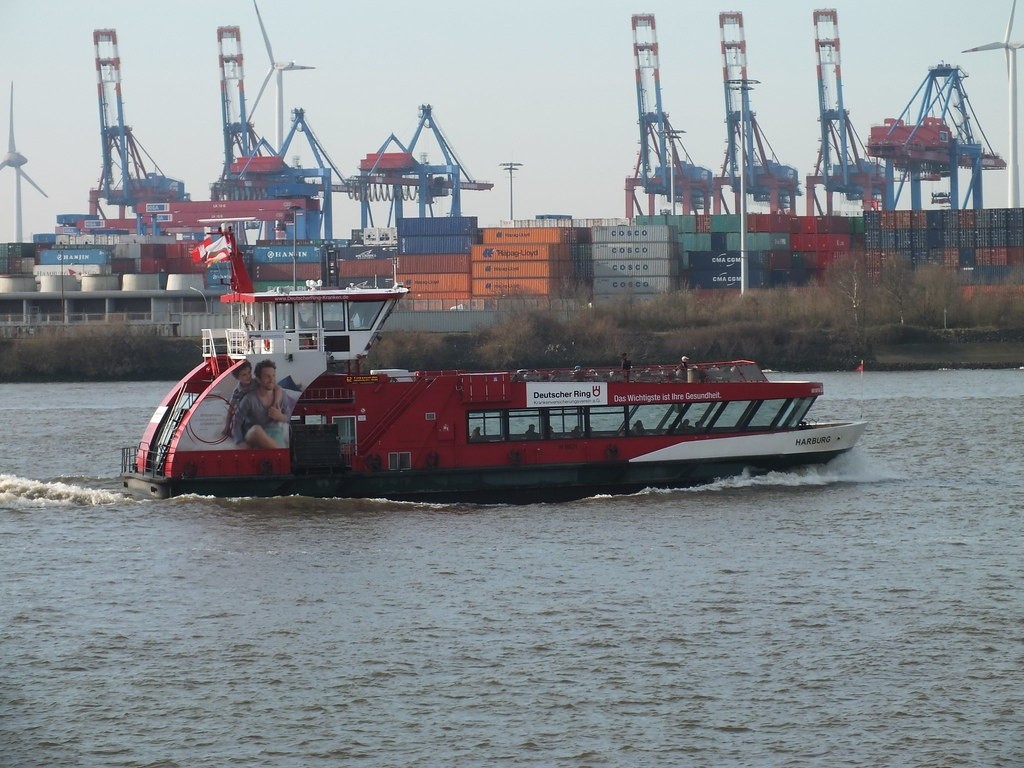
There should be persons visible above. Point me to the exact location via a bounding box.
[533,351,725,382]
[221,359,290,449]
[469,418,707,441]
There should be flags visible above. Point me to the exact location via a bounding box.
[190,234,233,266]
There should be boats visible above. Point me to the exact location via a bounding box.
[120,217,868,502]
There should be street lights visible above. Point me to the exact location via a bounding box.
[285,205,304,292]
[500,163,524,219]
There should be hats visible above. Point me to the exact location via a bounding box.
[682,356,689,362]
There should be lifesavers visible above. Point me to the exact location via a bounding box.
[303,336,317,350]
